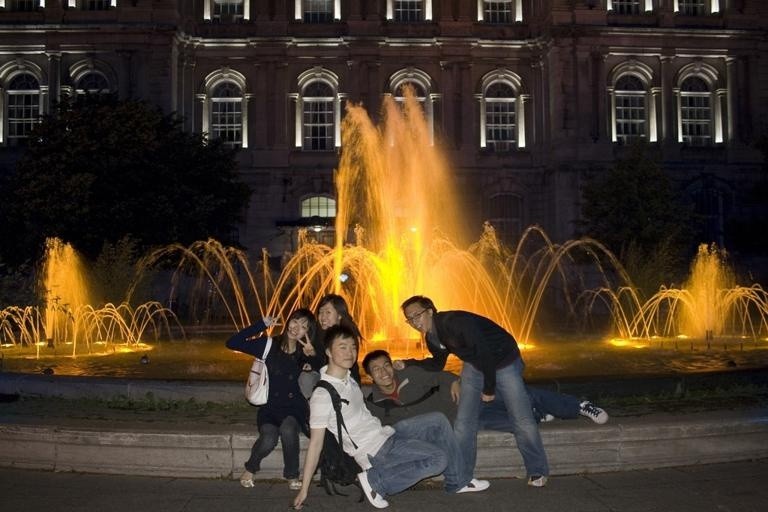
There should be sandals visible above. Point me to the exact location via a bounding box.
[287,478,302,490]
[241,471,255,488]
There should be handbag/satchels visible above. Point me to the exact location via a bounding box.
[244,336,273,406]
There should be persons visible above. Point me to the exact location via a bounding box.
[290,322,492,510]
[294,292,364,391]
[224,307,319,490]
[391,296,552,487]
[354,350,611,435]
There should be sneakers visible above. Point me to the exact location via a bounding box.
[357,471,389,509]
[528,473,547,487]
[578,400,609,424]
[456,478,490,493]
[539,413,554,423]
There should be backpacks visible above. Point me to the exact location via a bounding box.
[312,380,363,486]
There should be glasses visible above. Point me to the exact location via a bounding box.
[372,361,392,372]
[404,309,428,324]
